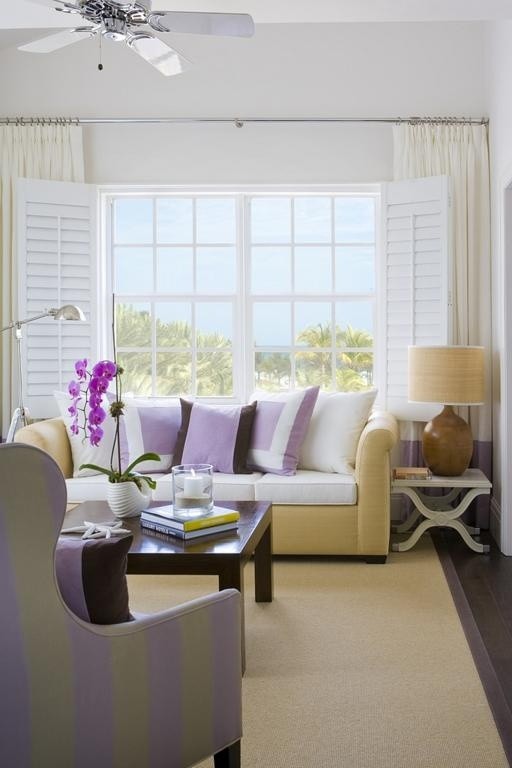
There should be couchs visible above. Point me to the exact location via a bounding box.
[14,407,399,564]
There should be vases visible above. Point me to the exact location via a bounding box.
[106,472,152,518]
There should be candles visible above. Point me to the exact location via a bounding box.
[184,468,203,497]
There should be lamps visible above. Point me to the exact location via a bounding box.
[0,304,87,428]
[406,345,485,476]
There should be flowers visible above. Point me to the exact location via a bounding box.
[65,292,161,490]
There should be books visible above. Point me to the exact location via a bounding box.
[141,499,242,550]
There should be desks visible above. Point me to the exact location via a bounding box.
[390,469,492,553]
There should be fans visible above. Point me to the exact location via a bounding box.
[0,0,254,77]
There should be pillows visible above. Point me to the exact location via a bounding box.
[245,388,322,478]
[55,535,136,625]
[297,388,379,476]
[54,388,120,478]
[111,397,185,476]
[164,397,260,477]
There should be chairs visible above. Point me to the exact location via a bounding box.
[0,441,244,768]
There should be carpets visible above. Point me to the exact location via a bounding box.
[126,520,512,768]
